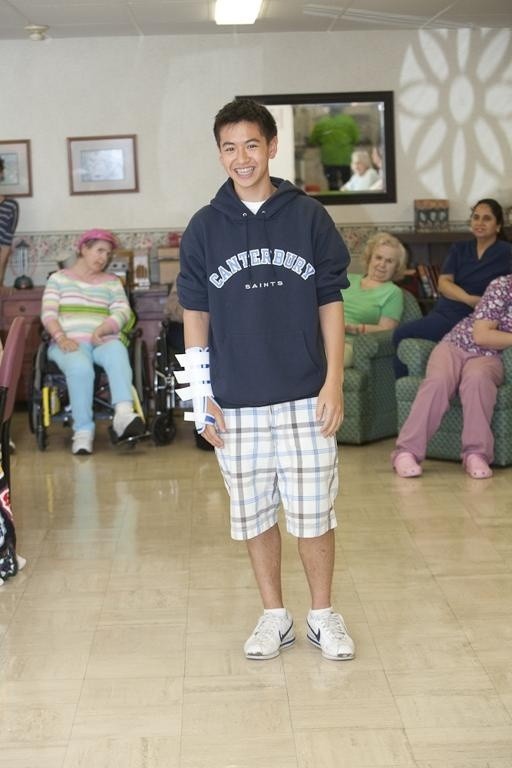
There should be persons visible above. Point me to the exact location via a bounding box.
[175,99,356,662]
[371,144,386,192]
[41,228,145,456]
[1,438,26,588]
[389,274,512,479]
[306,102,361,191]
[338,150,380,190]
[339,231,408,371]
[391,198,512,380]
[0,159,18,287]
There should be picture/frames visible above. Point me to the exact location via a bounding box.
[66,134,140,196]
[0,139,33,198]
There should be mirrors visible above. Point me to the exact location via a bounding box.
[234,90,397,205]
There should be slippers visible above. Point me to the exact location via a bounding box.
[461,453,493,479]
[393,452,422,478]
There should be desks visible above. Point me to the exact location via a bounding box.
[0,286,172,407]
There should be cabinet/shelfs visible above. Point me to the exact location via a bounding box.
[381,226,512,317]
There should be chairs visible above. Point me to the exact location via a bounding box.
[0,318,29,504]
[392,338,512,469]
[336,288,425,445]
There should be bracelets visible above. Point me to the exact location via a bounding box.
[361,323,366,334]
[50,328,65,341]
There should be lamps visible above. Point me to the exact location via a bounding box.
[13,240,35,289]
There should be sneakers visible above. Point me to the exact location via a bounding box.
[72,430,94,455]
[112,411,147,441]
[305,609,355,661]
[242,609,297,660]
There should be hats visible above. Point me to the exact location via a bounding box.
[79,230,118,249]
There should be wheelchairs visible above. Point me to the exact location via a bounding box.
[156,322,214,454]
[26,316,150,450]
